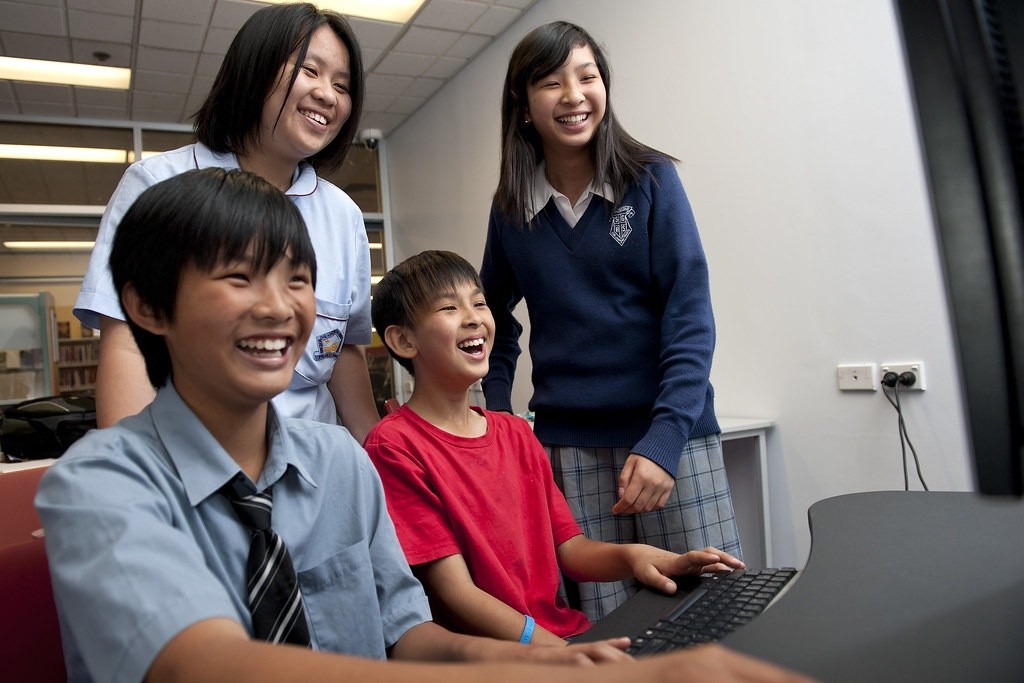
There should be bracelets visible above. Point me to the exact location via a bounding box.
[517,611,538,646]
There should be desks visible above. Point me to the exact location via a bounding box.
[519,411,775,567]
[717,491,1024,683]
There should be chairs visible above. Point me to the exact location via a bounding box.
[0,457,68,683]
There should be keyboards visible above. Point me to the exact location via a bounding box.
[566,566,798,661]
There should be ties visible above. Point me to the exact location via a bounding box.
[221,471,313,650]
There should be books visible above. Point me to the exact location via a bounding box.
[56,318,101,340]
[57,342,101,391]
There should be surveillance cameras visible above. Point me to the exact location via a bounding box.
[358,129,382,151]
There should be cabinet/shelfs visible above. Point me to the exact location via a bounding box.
[59,336,100,392]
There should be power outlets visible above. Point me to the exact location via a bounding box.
[838,364,877,392]
[881,362,927,391]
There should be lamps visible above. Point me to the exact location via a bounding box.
[0,143,162,164]
[0,202,106,251]
[0,56,133,93]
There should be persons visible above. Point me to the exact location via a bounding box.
[470,21,753,622]
[74,2,380,444]
[32,169,818,682]
[362,247,748,651]
